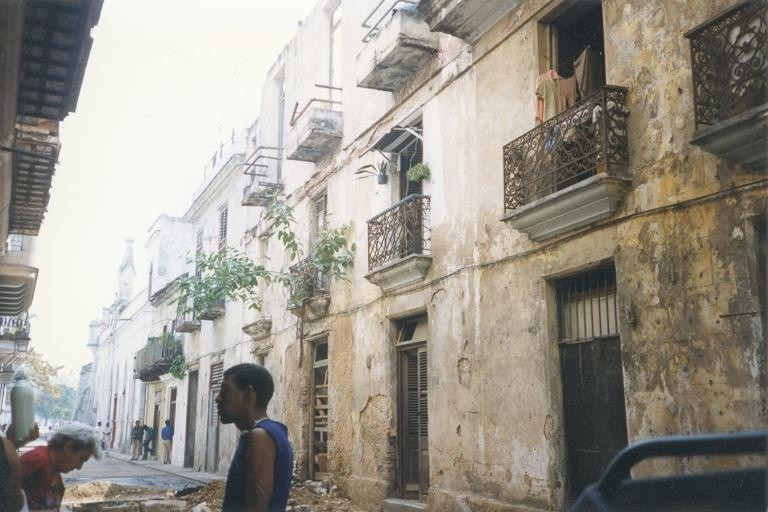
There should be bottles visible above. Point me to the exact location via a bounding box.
[11,371,34,444]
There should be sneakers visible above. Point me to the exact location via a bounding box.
[129,448,170,464]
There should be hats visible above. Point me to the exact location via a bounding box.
[59,422,100,460]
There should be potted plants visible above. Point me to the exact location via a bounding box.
[355,160,387,184]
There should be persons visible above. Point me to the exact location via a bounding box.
[46,427,55,441]
[93,421,112,457]
[0,437,28,511]
[215,363,294,512]
[18,422,102,511]
[162,419,174,465]
[129,420,143,460]
[141,425,154,460]
